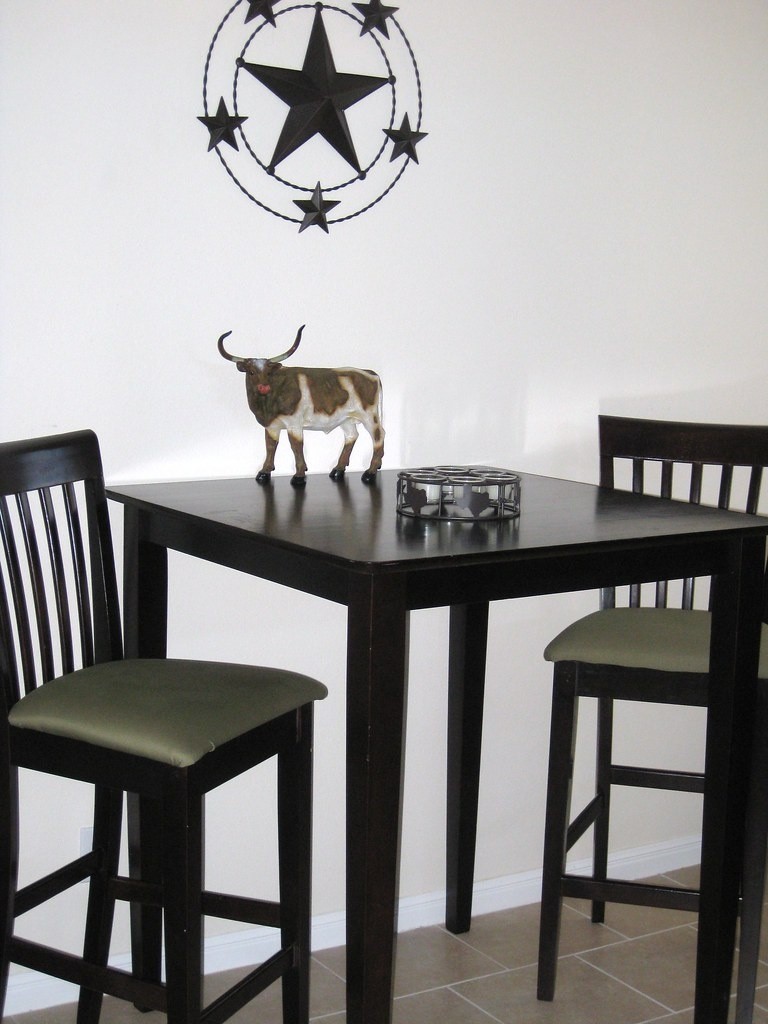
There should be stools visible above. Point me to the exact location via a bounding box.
[0,429,329,1024]
[537,416,768,1024]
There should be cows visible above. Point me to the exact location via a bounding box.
[218,325,386,485]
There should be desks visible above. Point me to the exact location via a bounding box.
[103,470,768,1023]
